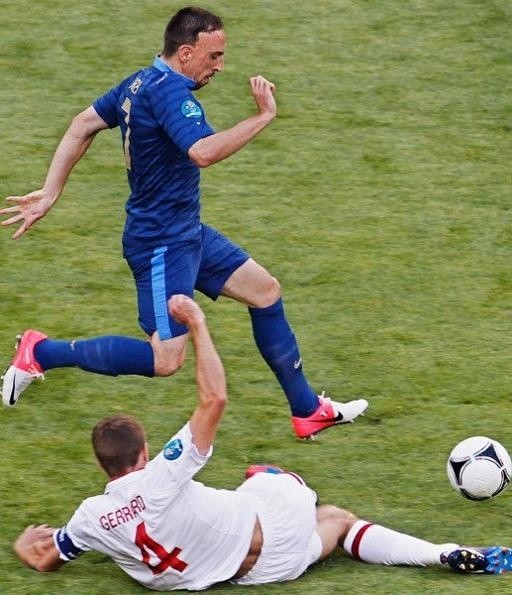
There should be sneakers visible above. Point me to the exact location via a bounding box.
[1,327,51,410]
[241,459,318,504]
[287,392,371,447]
[437,537,510,579]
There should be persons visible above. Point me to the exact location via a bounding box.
[0,8,370,440]
[13,292,509,593]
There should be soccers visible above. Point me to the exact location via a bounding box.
[446,435,512,501]
